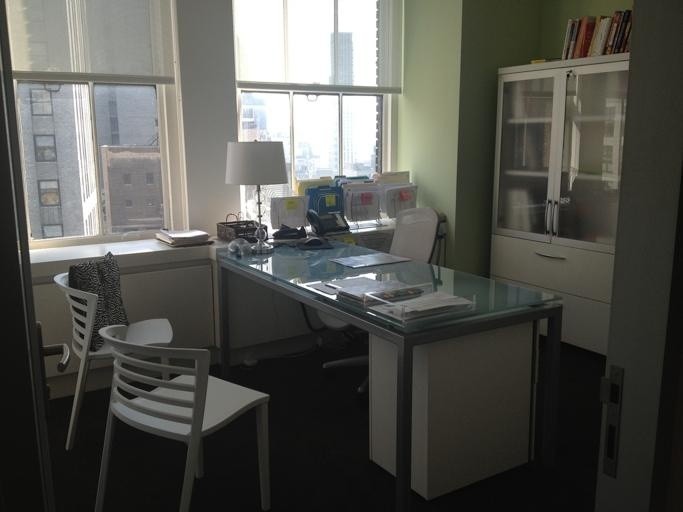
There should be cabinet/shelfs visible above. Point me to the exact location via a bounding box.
[488,52,630,358]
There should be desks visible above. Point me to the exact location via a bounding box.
[209,240,561,511]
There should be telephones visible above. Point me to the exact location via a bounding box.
[305,209,350,233]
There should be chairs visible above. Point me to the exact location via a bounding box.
[96,322,270,511]
[315,203,440,393]
[53,272,175,454]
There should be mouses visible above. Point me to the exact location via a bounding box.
[305,238,322,245]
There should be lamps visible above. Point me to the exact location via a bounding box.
[225,141,289,255]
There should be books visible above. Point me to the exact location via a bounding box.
[336,280,424,310]
[561,11,632,61]
[530,57,561,64]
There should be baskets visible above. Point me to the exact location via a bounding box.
[216,213,268,243]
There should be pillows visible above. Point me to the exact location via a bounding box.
[68,252,129,351]
